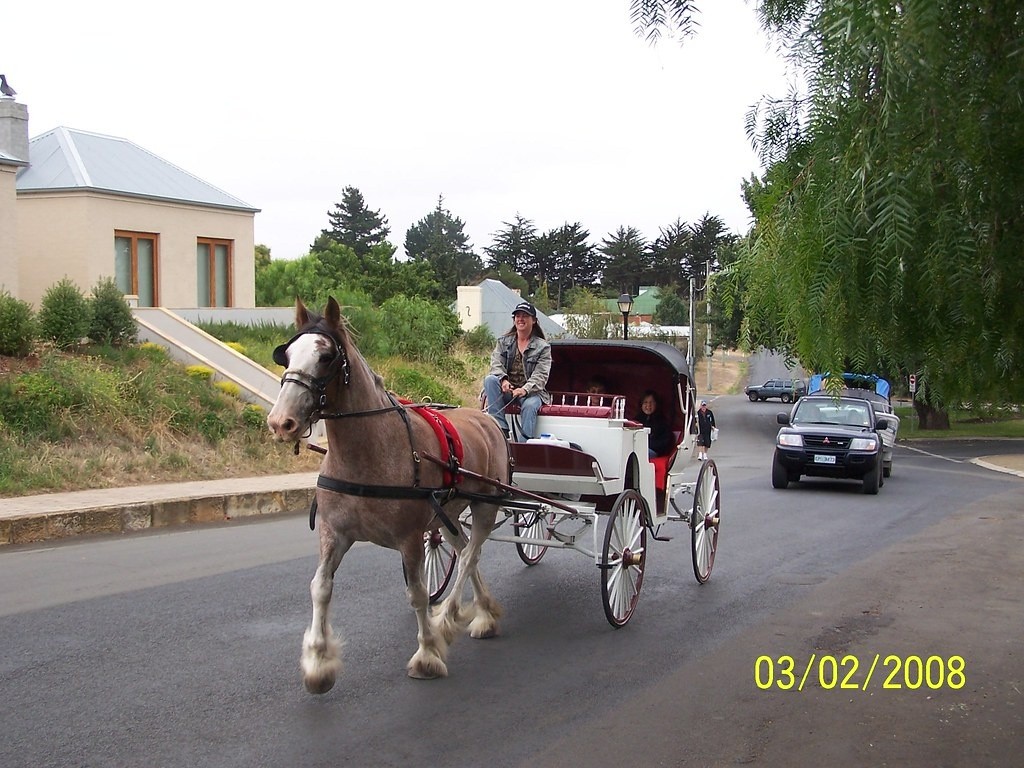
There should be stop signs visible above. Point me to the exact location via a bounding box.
[910,376,915,385]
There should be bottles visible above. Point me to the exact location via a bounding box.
[541,434,551,441]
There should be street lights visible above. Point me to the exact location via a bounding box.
[616,292,635,340]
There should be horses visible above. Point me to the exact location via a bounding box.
[266,294,513,694]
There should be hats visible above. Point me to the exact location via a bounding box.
[701,400,706,406]
[512,303,536,318]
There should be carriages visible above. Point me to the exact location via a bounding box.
[264,293,722,695]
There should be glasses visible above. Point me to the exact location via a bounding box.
[702,404,706,406]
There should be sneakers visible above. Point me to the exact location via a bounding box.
[698,456,702,461]
[704,455,708,460]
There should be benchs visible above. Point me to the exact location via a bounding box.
[545,362,692,492]
[481,391,628,427]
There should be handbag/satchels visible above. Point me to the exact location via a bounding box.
[710,426,719,441]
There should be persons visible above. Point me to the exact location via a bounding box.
[802,407,822,423]
[695,400,717,460]
[484,303,553,443]
[846,409,862,424]
[635,390,675,457]
[586,379,606,406]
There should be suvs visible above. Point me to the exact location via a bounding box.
[744,377,806,404]
[770,380,900,495]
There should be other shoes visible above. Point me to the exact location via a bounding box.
[502,428,510,438]
